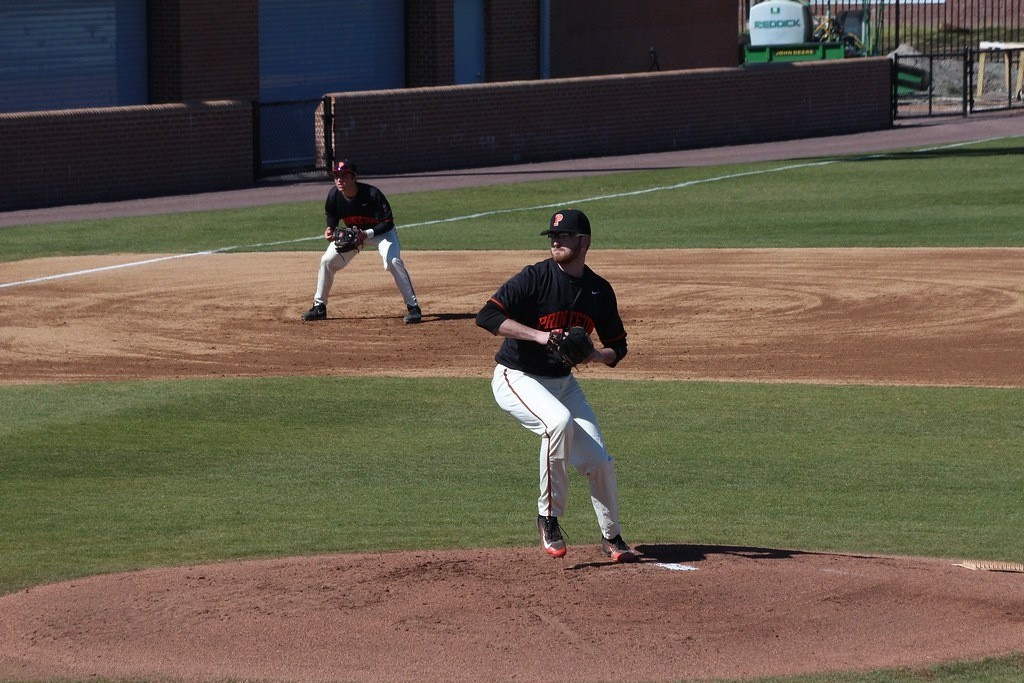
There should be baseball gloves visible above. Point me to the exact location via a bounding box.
[334,226,367,253]
[548,325,595,369]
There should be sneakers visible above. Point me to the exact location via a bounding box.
[602,535,636,562]
[301,303,326,320]
[538,515,569,559]
[403,305,421,322]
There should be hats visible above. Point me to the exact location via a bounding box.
[540,209,591,237]
[330,161,359,176]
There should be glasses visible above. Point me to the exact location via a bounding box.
[548,233,586,240]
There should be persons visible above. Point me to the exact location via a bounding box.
[300,161,422,323]
[475,207,635,560]
